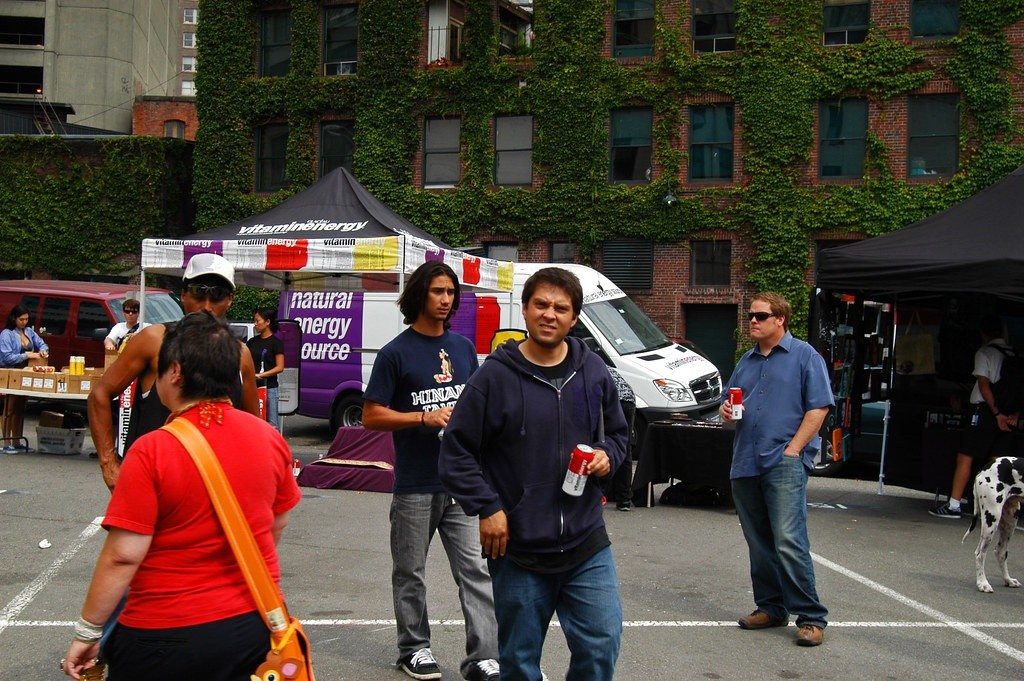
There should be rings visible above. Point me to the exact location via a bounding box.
[60,658,66,671]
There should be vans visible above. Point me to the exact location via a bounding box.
[276,263,724,463]
[0,279,188,427]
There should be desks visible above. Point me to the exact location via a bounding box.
[631,419,736,511]
[921,416,1024,513]
[0,388,119,459]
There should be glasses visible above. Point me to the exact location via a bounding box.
[184,277,232,305]
[124,310,138,314]
[748,312,778,321]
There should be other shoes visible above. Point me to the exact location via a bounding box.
[89,452,98,458]
[80,662,105,681]
[15,444,34,453]
[3,447,19,454]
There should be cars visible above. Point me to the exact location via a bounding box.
[228,322,260,344]
[802,364,891,477]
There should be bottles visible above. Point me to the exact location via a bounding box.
[69,355,85,375]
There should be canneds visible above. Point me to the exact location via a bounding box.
[292,459,301,476]
[727,387,742,419]
[562,444,595,496]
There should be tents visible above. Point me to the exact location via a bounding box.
[812,164,1024,470]
[138,166,515,441]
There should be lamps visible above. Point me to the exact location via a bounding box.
[663,177,676,204]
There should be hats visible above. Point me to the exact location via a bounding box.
[182,253,236,290]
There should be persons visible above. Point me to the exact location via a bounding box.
[439,268,628,681]
[363,260,501,681]
[0,305,48,454]
[928,315,1024,518]
[60,253,302,681]
[718,292,835,647]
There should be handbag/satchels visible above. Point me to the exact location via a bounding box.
[250,620,312,681]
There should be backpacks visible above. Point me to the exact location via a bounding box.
[987,343,1024,417]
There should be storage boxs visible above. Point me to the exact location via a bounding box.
[0,353,104,455]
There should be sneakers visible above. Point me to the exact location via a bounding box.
[461,658,500,681]
[400,647,442,679]
[739,609,788,630]
[797,624,823,645]
[927,502,962,519]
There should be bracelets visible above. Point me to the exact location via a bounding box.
[993,410,1001,417]
[74,616,103,644]
[422,412,426,427]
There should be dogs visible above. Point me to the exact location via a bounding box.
[962,455,1024,593]
